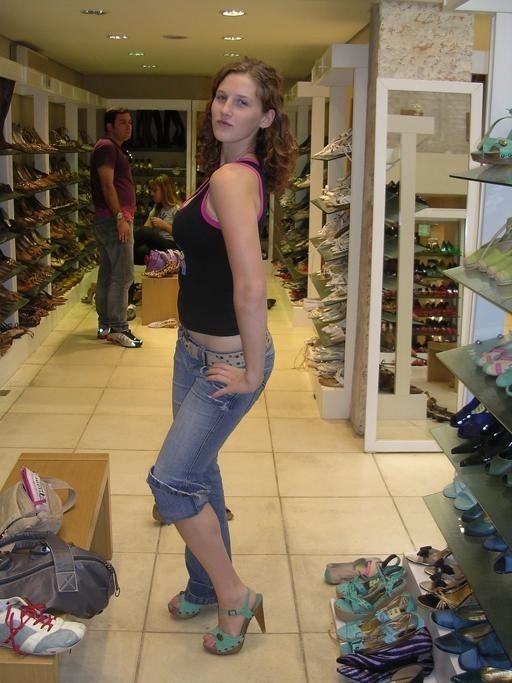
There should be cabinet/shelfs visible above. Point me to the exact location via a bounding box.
[396,0,511,683]
[1,52,120,391]
[381,178,463,385]
[265,80,330,306]
[310,42,370,423]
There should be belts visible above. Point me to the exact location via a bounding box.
[181,330,272,368]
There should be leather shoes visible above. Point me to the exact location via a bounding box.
[450,397,512,486]
[1,183,21,355]
[380,180,459,365]
[443,474,512,573]
[13,124,99,327]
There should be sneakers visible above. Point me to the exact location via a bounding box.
[271,135,311,306]
[97,328,110,339]
[108,330,143,347]
[475,341,512,395]
[375,368,454,421]
[1,598,86,654]
[303,299,346,341]
[462,216,512,286]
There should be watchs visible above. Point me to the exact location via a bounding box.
[115,211,122,220]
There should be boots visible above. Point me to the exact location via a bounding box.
[0,75,16,149]
[127,109,186,148]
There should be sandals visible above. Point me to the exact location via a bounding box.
[471,110,512,166]
[317,209,349,255]
[324,546,512,683]
[306,341,344,387]
[317,262,347,299]
[320,174,351,206]
[313,128,352,159]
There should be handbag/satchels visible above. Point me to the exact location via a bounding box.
[145,250,182,273]
[0,469,76,542]
[1,532,117,617]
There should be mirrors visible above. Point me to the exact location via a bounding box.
[358,73,489,456]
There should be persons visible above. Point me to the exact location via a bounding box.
[146,58,299,657]
[134,174,184,264]
[88,107,144,348]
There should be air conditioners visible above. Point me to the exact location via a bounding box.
[10,43,49,74]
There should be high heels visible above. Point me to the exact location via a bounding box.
[203,589,265,656]
[169,592,219,618]
[149,319,176,328]
[142,262,176,278]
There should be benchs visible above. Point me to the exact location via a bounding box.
[0,445,119,682]
[139,270,180,328]
